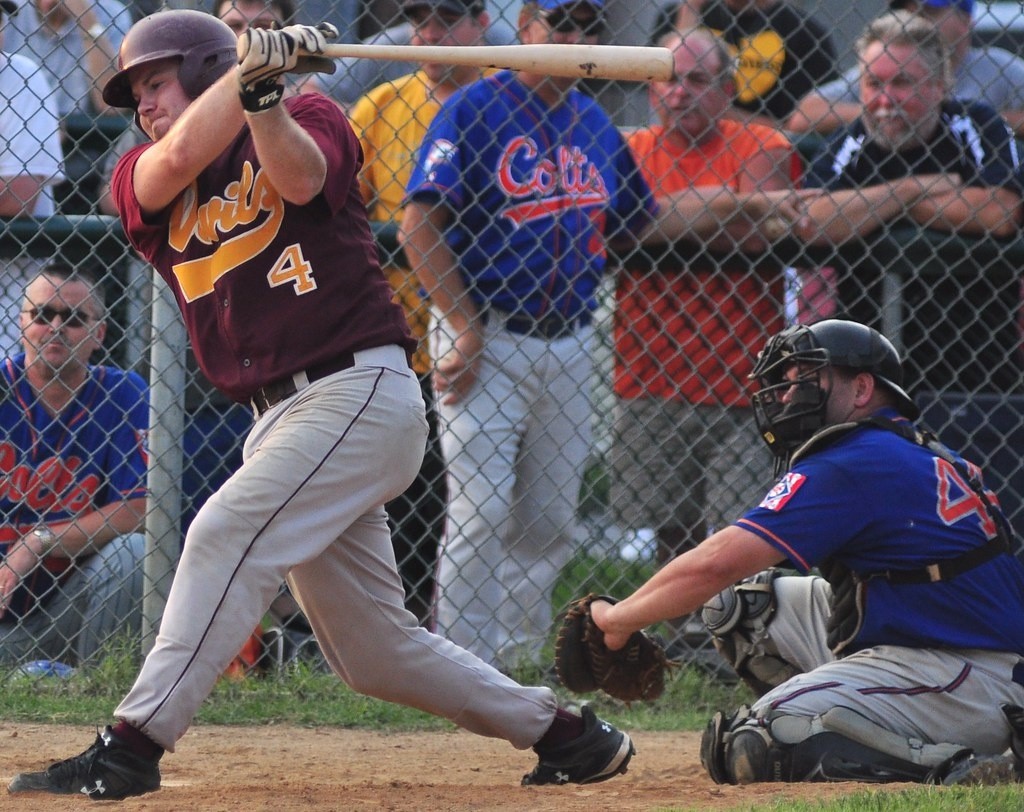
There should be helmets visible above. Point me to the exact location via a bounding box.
[809,317,918,422]
[100,10,240,140]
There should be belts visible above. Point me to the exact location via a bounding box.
[251,350,415,417]
[477,308,592,340]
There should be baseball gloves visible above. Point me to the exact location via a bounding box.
[555,593,667,702]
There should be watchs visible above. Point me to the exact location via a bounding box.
[34,527,52,558]
[80,22,107,40]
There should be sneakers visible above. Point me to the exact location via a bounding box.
[520,705,637,785]
[7,723,162,800]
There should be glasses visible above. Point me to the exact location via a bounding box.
[536,8,606,36]
[21,303,106,328]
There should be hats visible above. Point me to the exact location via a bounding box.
[889,0,974,12]
[523,0,612,15]
[399,0,485,14]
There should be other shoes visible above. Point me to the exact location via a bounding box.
[938,748,1013,786]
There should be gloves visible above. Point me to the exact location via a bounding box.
[283,21,339,75]
[236,21,297,115]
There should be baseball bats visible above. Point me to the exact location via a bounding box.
[235,33,675,83]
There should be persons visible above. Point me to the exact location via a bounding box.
[607,0,1024,684]
[0,0,506,680]
[7,10,637,801]
[0,260,151,674]
[553,318,1024,784]
[397,0,660,672]
